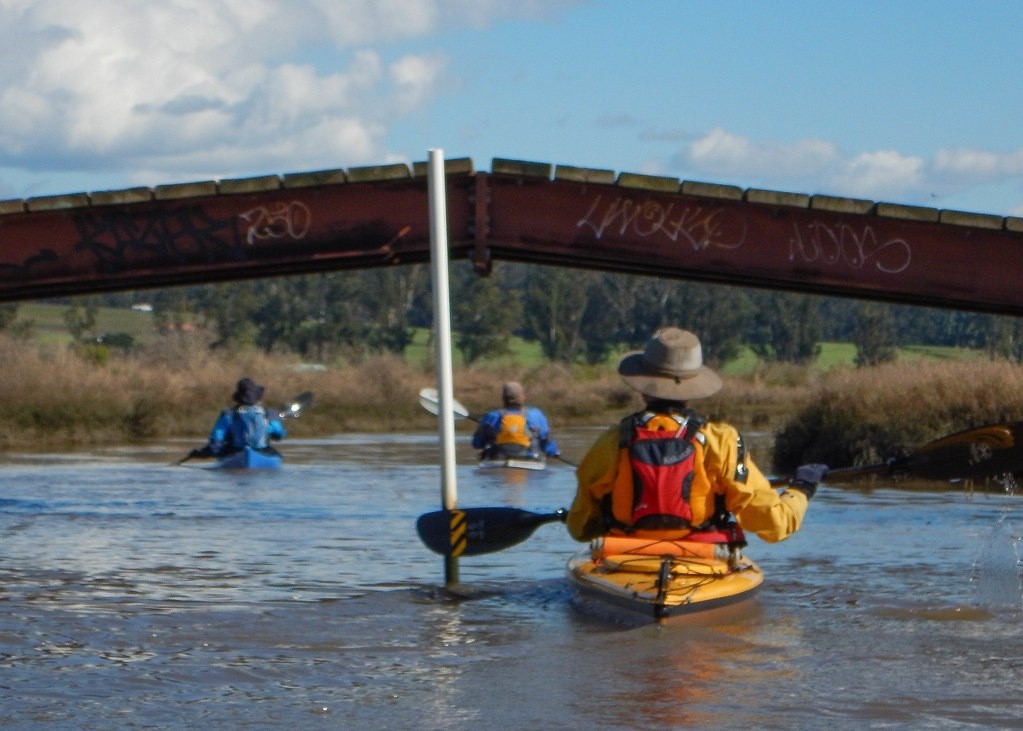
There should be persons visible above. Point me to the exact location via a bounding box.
[188,377,286,458]
[567,326,825,562]
[471,382,561,462]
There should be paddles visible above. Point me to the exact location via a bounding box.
[419,389,578,468]
[417,420,1023,558]
[166,391,315,465]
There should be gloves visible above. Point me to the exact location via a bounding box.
[794,462,828,497]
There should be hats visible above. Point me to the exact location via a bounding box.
[618,327,723,402]
[232,377,264,405]
[503,382,524,404]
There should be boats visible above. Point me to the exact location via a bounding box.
[202,446,281,472]
[564,539,766,623]
[475,452,548,471]
